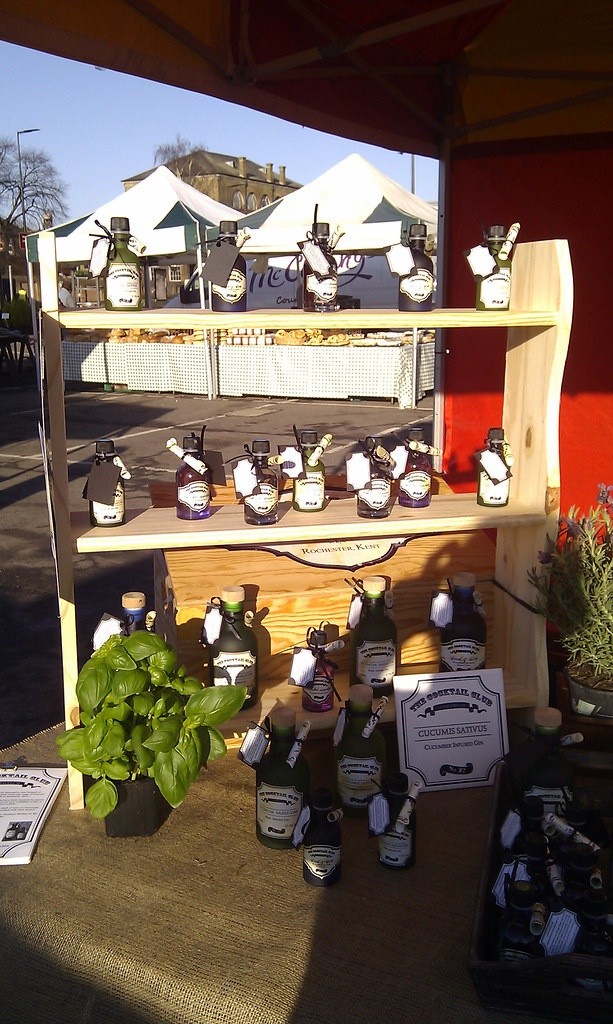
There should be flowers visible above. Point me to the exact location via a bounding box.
[526,485,613,675]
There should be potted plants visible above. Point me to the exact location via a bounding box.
[55,630,247,838]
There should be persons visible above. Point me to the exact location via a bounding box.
[59,280,76,308]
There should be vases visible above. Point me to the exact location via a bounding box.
[560,664,613,719]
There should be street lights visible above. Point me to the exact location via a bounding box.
[17,128,41,234]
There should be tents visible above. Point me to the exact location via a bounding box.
[24,164,245,393]
[208,154,440,411]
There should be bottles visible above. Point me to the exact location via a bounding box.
[175,435,211,521]
[292,430,325,513]
[302,223,338,313]
[476,427,510,507]
[398,427,432,508]
[301,631,334,712]
[103,217,143,311]
[243,439,279,525]
[492,707,613,993]
[476,225,512,311]
[357,434,393,520]
[121,591,147,634]
[89,439,126,527]
[253,685,416,887]
[209,586,258,710]
[439,571,487,673]
[398,224,433,312]
[211,220,247,312]
[349,577,398,697]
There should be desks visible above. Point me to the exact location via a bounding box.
[0,718,613,1024]
[149,476,496,682]
[61,339,435,408]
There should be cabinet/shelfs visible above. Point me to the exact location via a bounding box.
[34,229,575,816]
